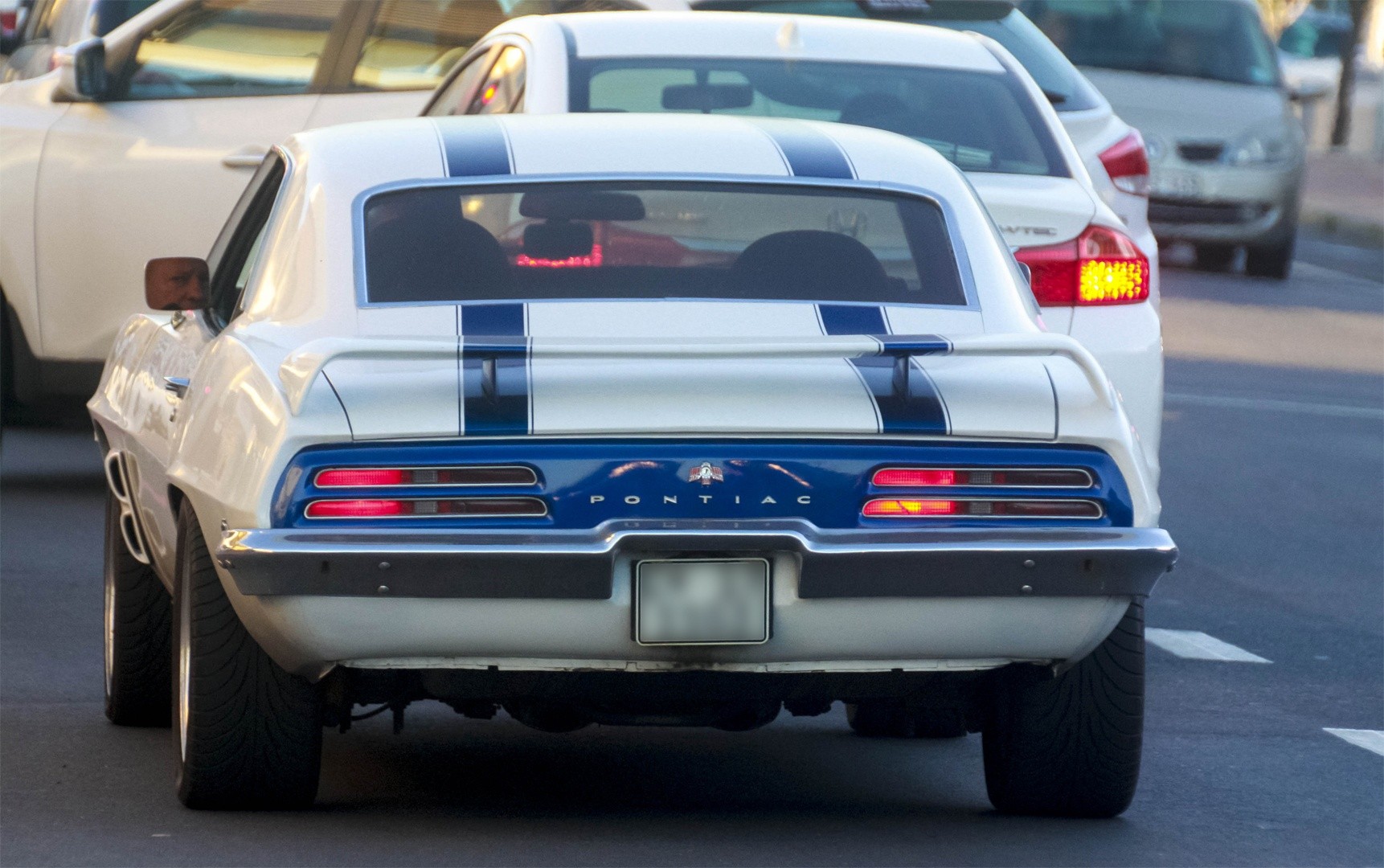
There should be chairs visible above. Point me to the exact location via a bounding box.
[366,216,523,303]
[838,93,915,138]
[725,230,893,302]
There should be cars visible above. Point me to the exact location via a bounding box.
[416,13,1164,739]
[0,2,157,88]
[84,109,1181,825]
[1013,0,1345,277]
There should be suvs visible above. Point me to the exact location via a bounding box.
[0,1,1165,434]
